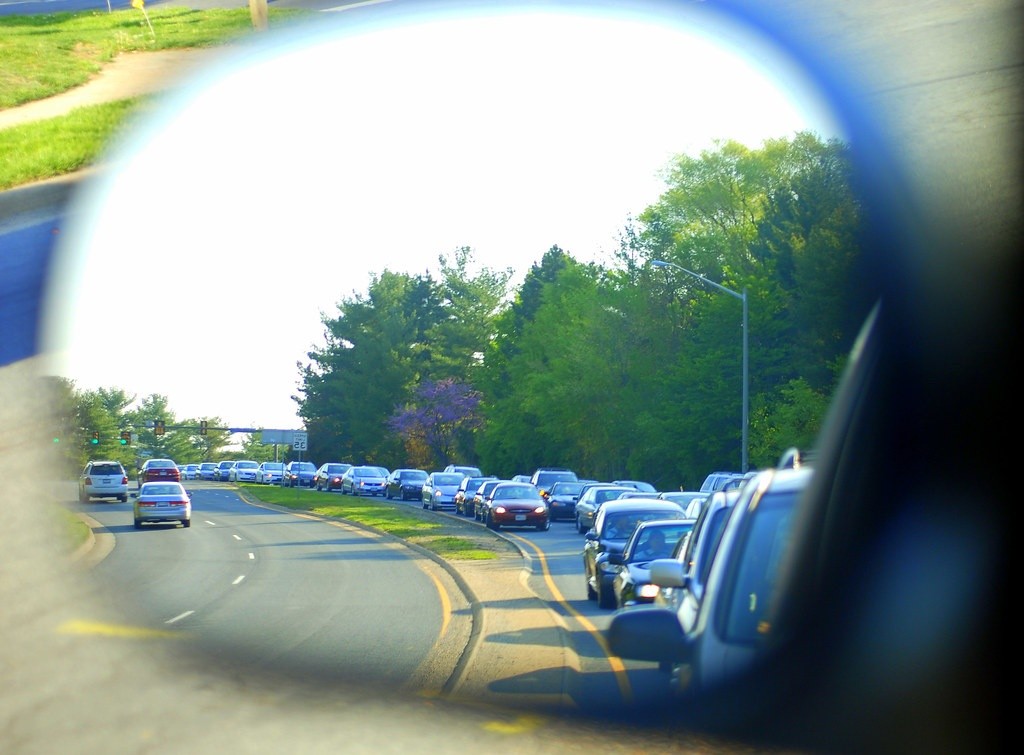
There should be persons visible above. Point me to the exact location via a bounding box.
[633,530,672,563]
[605,515,630,539]
[559,486,570,494]
[596,493,608,503]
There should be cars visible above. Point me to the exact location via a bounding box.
[134,481,191,528]
[314,462,352,492]
[342,465,389,496]
[441,466,814,696]
[422,471,467,510]
[182,460,261,482]
[79,460,128,501]
[281,461,319,486]
[382,470,434,500]
[473,482,516,518]
[486,482,550,532]
[455,477,497,515]
[139,459,180,488]
[255,462,291,485]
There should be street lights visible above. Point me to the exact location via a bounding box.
[652,258,751,473]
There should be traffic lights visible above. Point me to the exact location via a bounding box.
[53,437,59,444]
[92,431,100,444]
[120,431,131,445]
